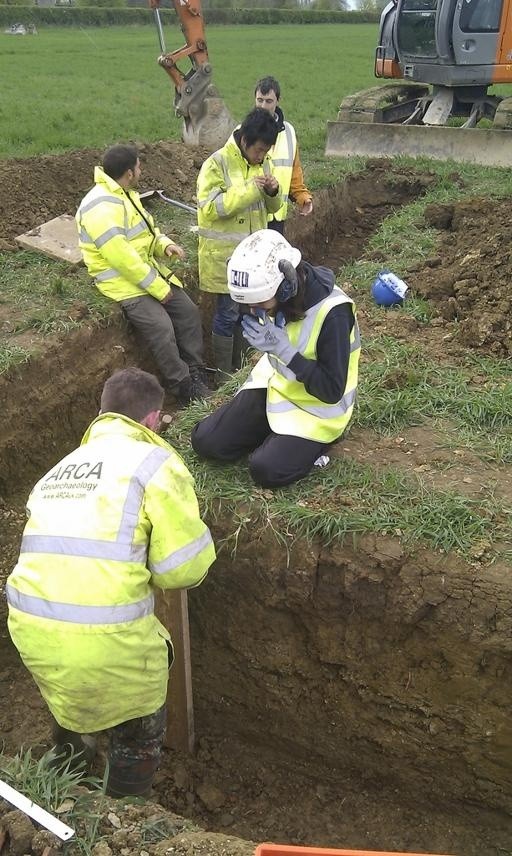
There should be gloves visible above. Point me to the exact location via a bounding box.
[241,309,298,367]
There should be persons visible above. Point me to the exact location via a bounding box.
[4,367,219,803]
[189,224,362,487]
[225,75,316,239]
[194,107,287,387]
[75,143,207,406]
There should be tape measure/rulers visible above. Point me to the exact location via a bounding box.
[0,780,76,841]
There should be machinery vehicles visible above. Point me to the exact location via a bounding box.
[149,1,511,168]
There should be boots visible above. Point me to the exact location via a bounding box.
[169,362,216,410]
[105,756,160,800]
[212,327,242,389]
[59,736,95,779]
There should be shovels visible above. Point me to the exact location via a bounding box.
[139,188,196,213]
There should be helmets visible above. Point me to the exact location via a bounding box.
[372,272,405,306]
[227,228,302,307]
